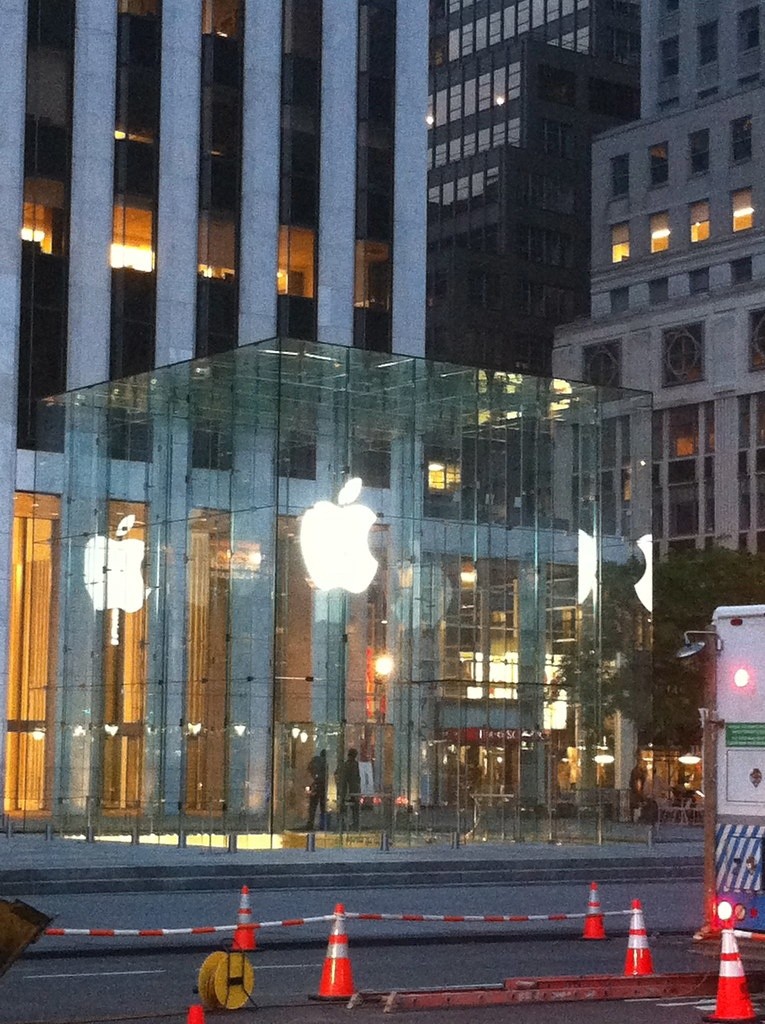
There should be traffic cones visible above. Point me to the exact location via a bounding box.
[696,911,765,1024]
[621,898,654,975]
[579,880,611,941]
[307,902,356,1000]
[229,886,260,952]
[186,1005,206,1024]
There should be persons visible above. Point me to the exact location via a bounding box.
[629,757,648,791]
[307,749,329,830]
[674,776,696,802]
[334,748,362,818]
